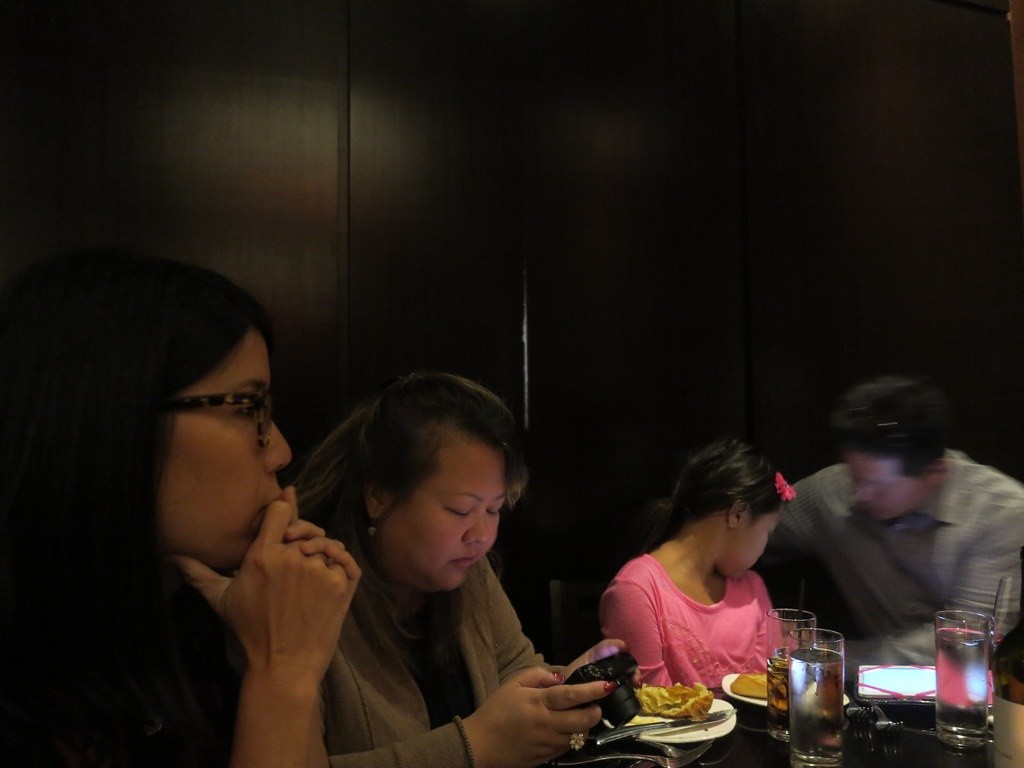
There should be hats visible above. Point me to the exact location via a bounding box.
[829,376,956,450]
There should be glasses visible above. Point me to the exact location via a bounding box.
[163,388,278,446]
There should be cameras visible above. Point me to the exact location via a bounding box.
[563,651,641,730]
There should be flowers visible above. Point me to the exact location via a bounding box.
[775,471,797,502]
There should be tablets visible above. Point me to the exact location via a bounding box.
[858,665,993,706]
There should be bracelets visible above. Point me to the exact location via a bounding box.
[453,715,475,766]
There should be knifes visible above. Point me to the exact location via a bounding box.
[596,709,738,745]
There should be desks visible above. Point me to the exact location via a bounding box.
[537,681,996,768]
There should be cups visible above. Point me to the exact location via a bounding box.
[788,628,844,768]
[935,610,990,751]
[990,576,1022,650]
[765,609,817,742]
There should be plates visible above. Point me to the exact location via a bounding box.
[722,673,850,706]
[625,698,736,743]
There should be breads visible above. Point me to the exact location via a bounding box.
[731,673,767,700]
[635,681,713,719]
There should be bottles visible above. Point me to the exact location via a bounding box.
[990,547,1024,768]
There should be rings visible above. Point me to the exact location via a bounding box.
[568,731,585,751]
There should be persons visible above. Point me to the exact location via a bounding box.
[766,374,1024,683]
[600,435,785,688]
[992,623,1024,705]
[284,372,639,768]
[0,261,360,768]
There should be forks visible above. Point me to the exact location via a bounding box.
[586,734,717,758]
[843,692,874,718]
[872,703,904,732]
[552,743,711,768]
